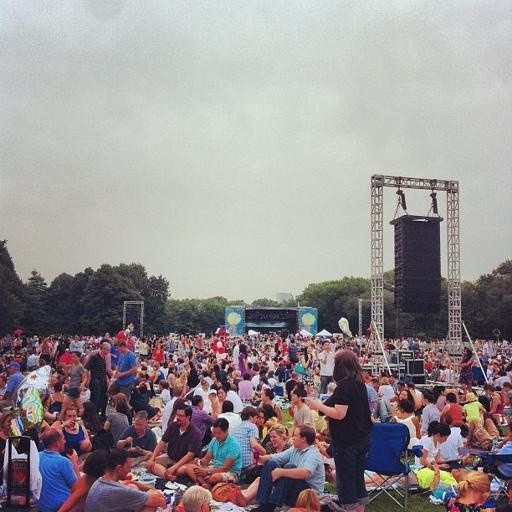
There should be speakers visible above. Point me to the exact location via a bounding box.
[127,304,141,337]
[393,222,441,314]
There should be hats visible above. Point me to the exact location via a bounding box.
[379,377,390,384]
[464,392,478,403]
[464,345,474,353]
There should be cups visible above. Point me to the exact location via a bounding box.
[195,458,200,466]
[308,392,318,411]
[69,421,76,431]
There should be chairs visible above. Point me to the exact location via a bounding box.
[364,423,410,509]
[472,365,487,386]
[486,454,512,504]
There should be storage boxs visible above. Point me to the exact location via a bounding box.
[405,359,425,375]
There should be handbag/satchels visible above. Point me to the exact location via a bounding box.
[294,362,307,375]
[210,481,241,503]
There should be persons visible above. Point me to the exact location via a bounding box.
[0,324,512,512]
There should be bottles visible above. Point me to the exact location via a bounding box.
[65,446,74,455]
